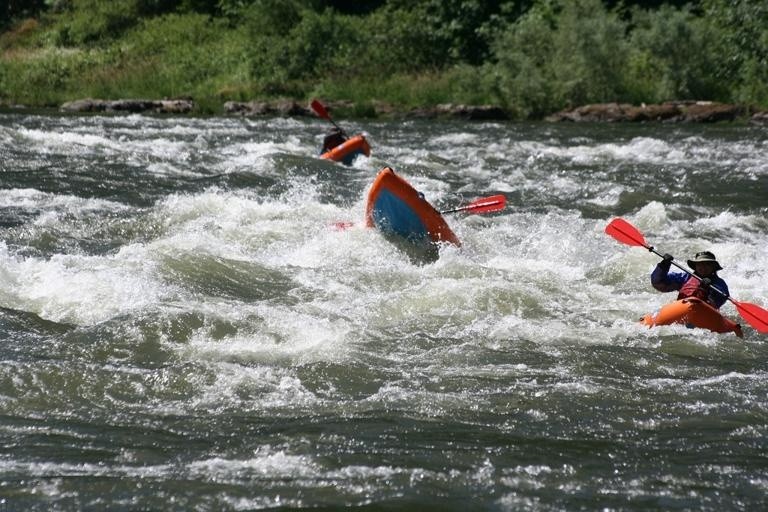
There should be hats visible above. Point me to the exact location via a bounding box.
[687,251,723,270]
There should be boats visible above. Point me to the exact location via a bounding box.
[640,296,743,339]
[365,167,475,268]
[318,134,371,167]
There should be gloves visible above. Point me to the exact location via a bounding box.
[662,253,674,266]
[700,278,712,289]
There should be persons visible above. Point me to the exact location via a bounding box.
[650,252,730,309]
[323,126,345,151]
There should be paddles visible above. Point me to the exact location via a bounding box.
[605,218,768,333]
[311,99,348,140]
[330,195,505,231]
